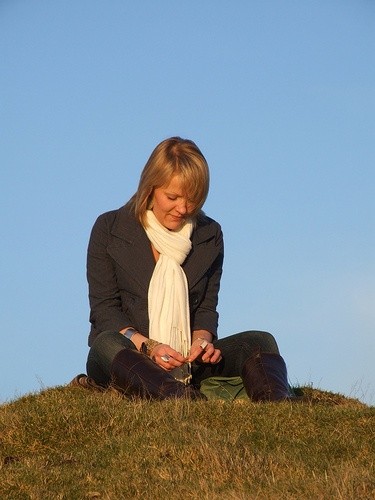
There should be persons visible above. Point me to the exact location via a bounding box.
[86,137,291,404]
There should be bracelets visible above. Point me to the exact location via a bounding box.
[139,339,162,356]
[124,328,138,339]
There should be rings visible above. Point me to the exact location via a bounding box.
[199,343,207,349]
[160,353,171,362]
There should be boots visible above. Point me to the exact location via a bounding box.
[108,347,208,403]
[241,351,312,403]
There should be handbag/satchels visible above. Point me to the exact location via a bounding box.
[200,376,250,401]
